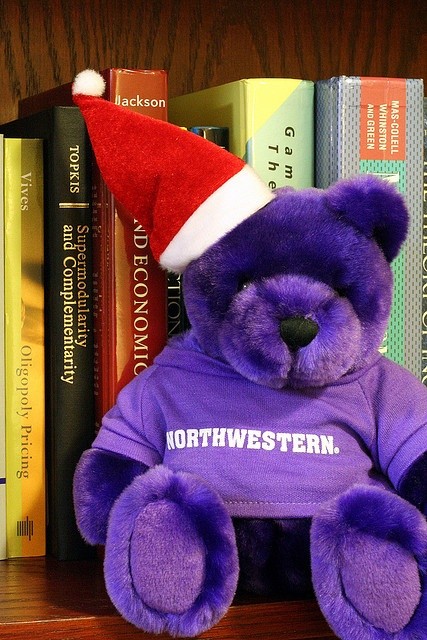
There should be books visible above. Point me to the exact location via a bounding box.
[1,137,47,559]
[2,107,94,559]
[168,79,316,341]
[315,76,425,382]
[18,68,169,446]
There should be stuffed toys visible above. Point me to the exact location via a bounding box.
[71,174,426,640]
